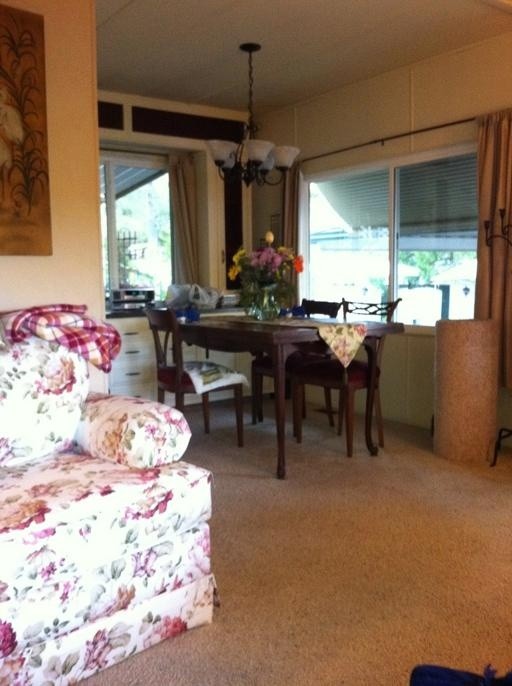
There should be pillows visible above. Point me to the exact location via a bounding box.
[1,333,89,472]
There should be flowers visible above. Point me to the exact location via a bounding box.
[229,231,303,318]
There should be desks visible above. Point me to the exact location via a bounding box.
[177,311,405,477]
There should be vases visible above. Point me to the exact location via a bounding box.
[245,279,281,320]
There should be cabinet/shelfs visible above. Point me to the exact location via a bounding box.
[107,311,274,406]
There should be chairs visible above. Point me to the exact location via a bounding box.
[288,297,402,459]
[143,306,243,448]
[1,331,222,686]
[250,297,342,424]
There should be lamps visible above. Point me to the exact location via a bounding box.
[205,42,300,188]
